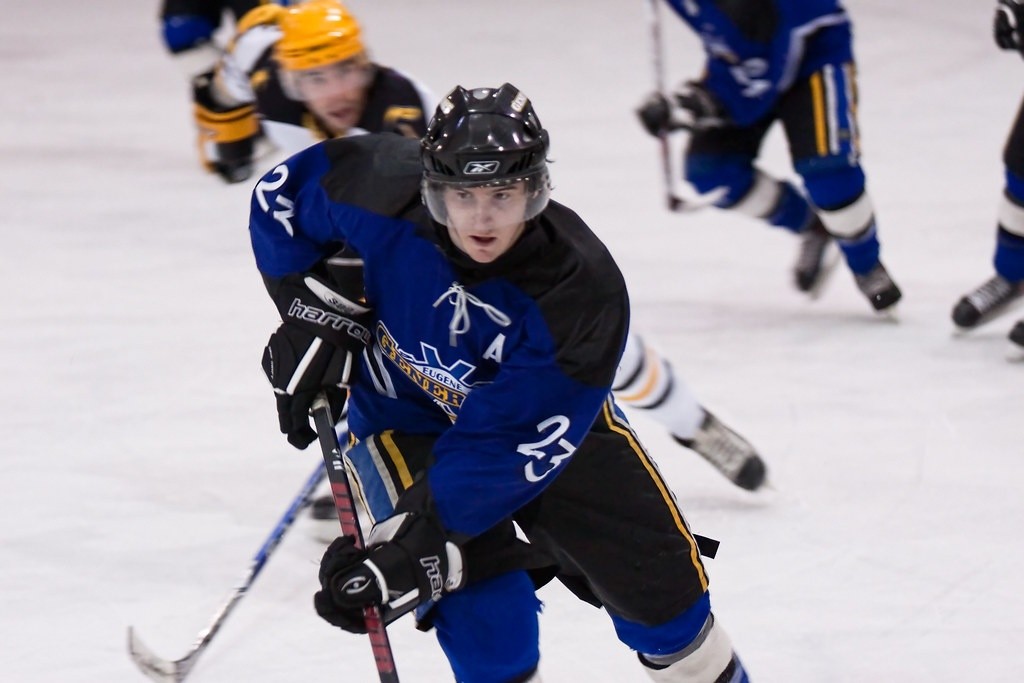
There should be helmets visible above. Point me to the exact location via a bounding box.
[419,83,553,231]
[272,0,373,97]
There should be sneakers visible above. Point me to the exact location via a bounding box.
[670,404,775,495]
[853,260,903,320]
[1004,322,1024,362]
[949,275,1024,339]
[793,220,842,298]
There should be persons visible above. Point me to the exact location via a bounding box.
[160,0,300,164]
[192,0,775,542]
[250,83,749,683]
[952,0,1024,360]
[637,0,901,314]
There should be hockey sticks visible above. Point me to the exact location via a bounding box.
[124,431,351,683]
[648,0,706,215]
[310,397,400,682]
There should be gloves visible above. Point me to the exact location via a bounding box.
[314,505,462,634]
[637,79,724,134]
[263,278,373,452]
[188,76,276,185]
